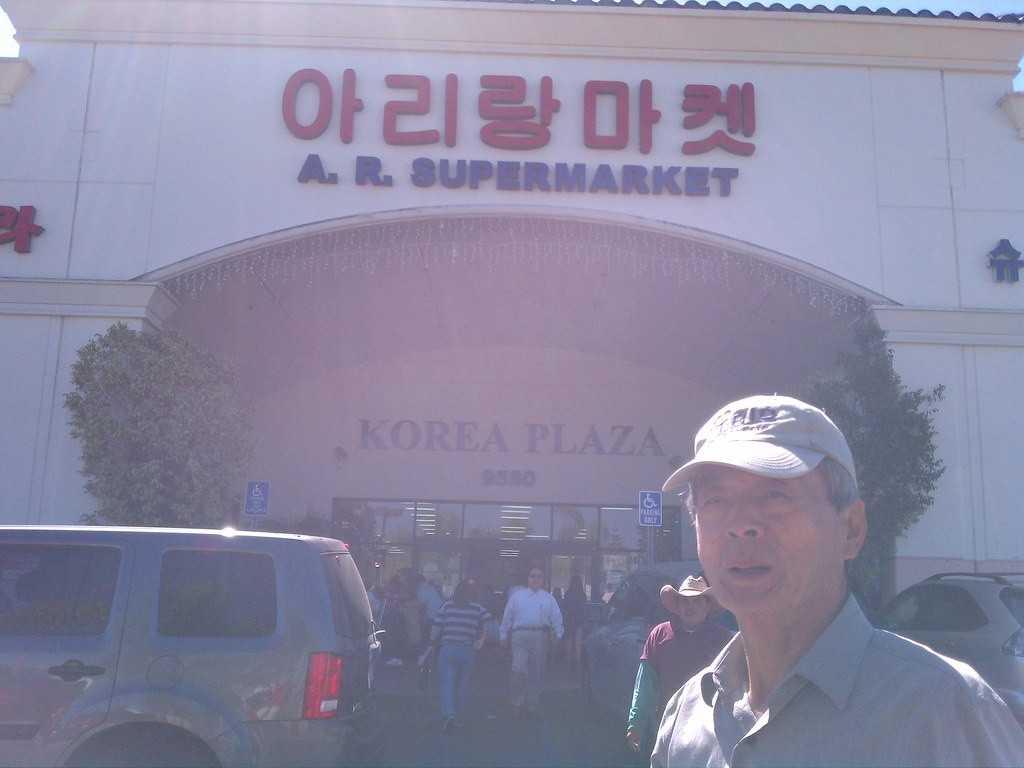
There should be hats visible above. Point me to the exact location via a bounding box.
[420,563,447,583]
[660,392,857,493]
[659,576,725,618]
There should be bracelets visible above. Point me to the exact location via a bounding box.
[428,639,435,646]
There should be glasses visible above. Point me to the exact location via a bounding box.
[530,574,543,579]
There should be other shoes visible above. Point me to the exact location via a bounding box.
[442,716,466,735]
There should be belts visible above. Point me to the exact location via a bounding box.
[515,627,548,631]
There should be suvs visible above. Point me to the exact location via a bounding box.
[0,524,379,768]
[861,572,1024,722]
[570,555,742,721]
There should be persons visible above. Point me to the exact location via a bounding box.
[427,583,495,735]
[380,569,442,666]
[563,575,587,664]
[587,575,734,752]
[499,568,564,720]
[648,394,1024,768]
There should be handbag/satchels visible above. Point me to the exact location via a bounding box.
[419,650,434,690]
